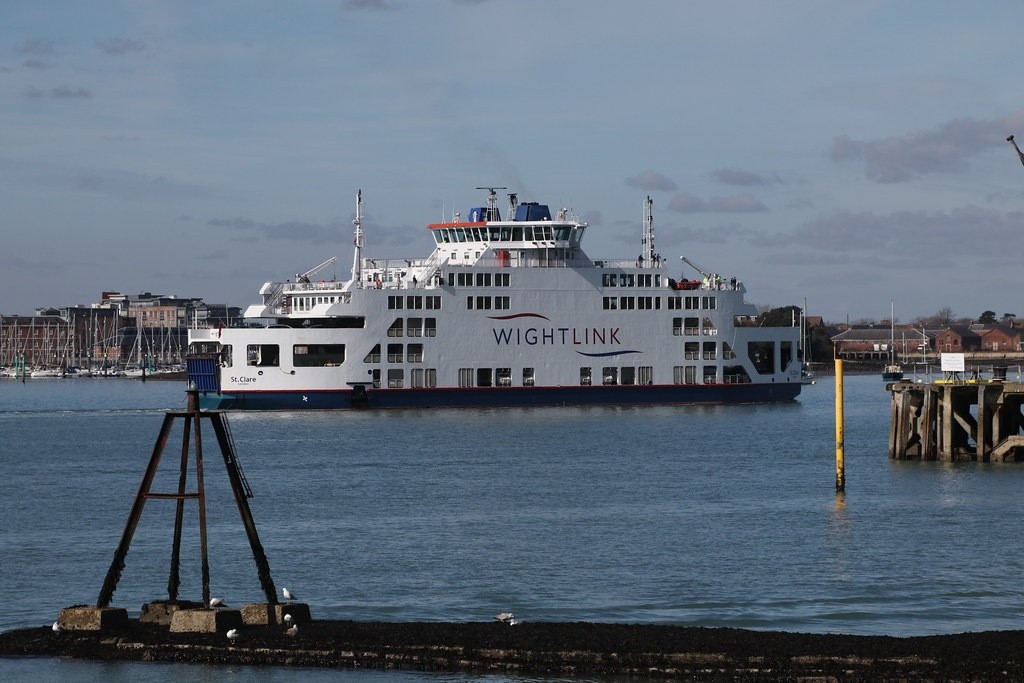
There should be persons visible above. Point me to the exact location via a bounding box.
[799,363,815,378]
[295,273,313,289]
[355,277,364,291]
[375,276,382,289]
[412,274,418,289]
[743,377,750,383]
[404,259,410,269]
[637,254,645,269]
[652,253,662,269]
[703,272,721,290]
[395,273,402,289]
[731,277,736,291]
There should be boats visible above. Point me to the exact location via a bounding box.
[186,187,817,410]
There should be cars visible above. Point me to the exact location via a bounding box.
[918,345,932,351]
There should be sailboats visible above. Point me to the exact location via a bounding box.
[0,300,187,378]
[882,300,903,381]
